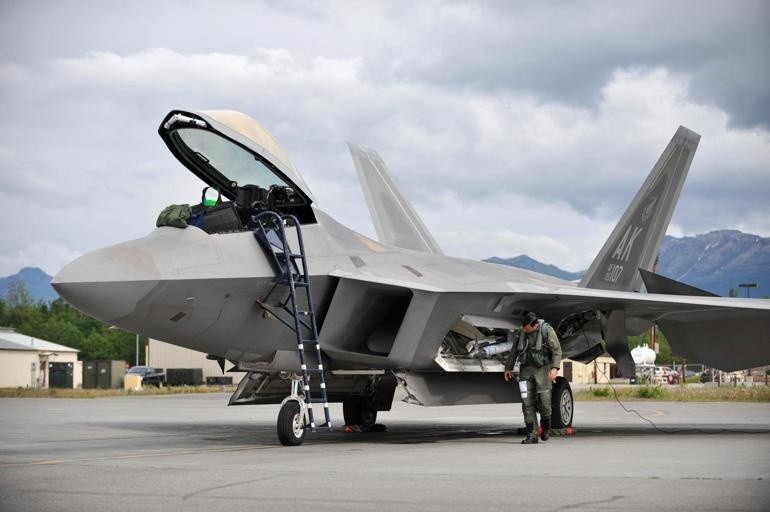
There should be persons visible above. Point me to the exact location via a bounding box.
[504,312,562,444]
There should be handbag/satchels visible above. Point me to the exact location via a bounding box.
[156,204,191,228]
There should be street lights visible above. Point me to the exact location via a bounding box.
[738,284,759,296]
[109,325,139,367]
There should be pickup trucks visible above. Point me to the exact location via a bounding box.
[126,365,166,389]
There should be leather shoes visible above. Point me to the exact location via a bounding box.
[521,438,538,444]
[541,428,549,440]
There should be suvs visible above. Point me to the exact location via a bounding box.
[656,367,679,384]
[701,369,717,383]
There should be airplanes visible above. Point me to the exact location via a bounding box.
[50,107,770,446]
